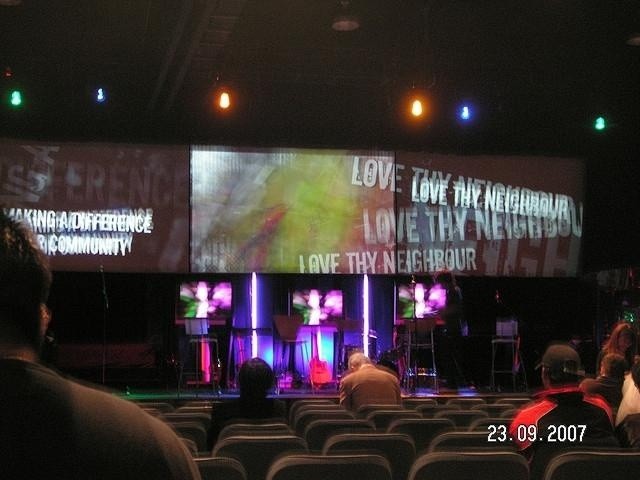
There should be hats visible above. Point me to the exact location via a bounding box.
[536,345,586,375]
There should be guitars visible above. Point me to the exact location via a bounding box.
[310,334,333,386]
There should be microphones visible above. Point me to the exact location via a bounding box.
[99,265,104,283]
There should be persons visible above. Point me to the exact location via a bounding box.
[507,341,616,456]
[581,320,640,448]
[206,356,287,449]
[0,209,205,480]
[337,352,402,414]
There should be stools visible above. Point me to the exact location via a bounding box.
[179,339,221,397]
[488,338,530,393]
[276,340,316,395]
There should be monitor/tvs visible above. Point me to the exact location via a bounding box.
[287,284,346,327]
[393,278,451,321]
[176,276,234,321]
